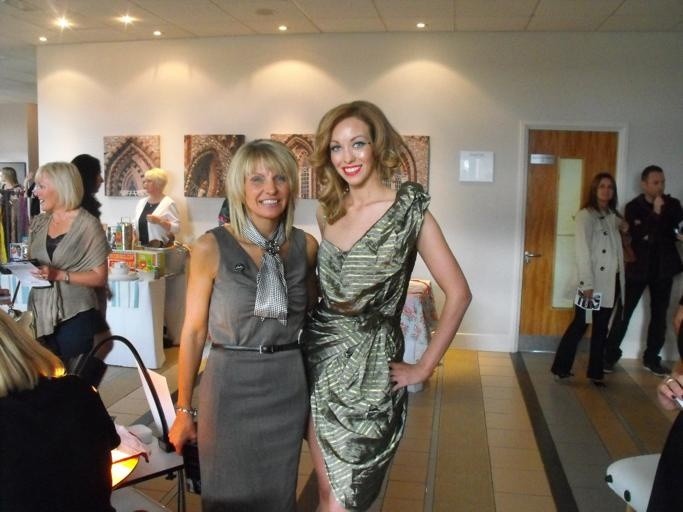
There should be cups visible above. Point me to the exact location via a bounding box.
[148,214,153,223]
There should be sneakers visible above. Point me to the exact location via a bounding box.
[641,352,672,379]
[551,370,573,386]
[587,360,617,388]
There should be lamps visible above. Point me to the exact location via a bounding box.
[53,334,176,457]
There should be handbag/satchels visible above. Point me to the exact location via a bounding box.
[621,231,636,263]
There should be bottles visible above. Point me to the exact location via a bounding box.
[108,223,132,250]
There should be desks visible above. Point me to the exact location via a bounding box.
[397,279,442,392]
[107,422,190,512]
[0,248,189,370]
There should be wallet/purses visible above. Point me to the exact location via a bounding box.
[179,439,203,496]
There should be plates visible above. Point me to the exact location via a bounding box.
[148,246,175,251]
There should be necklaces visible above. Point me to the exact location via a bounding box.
[52,220,65,226]
[150,204,153,208]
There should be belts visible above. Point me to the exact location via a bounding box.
[212,341,303,355]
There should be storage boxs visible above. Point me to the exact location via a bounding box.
[107,247,190,277]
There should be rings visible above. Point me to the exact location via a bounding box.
[666,378,673,383]
[40,274,42,279]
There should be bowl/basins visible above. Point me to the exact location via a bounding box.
[115,262,127,268]
[111,267,131,276]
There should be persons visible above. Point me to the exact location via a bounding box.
[12,245,19,258]
[312,101,472,512]
[72,156,112,388]
[26,172,39,217]
[552,172,630,387]
[23,163,112,372]
[646,295,683,512]
[3,169,23,191]
[137,168,180,249]
[603,164,683,372]
[168,138,319,512]
[0,302,121,512]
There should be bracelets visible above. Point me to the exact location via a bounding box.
[64,271,68,283]
[174,408,197,417]
